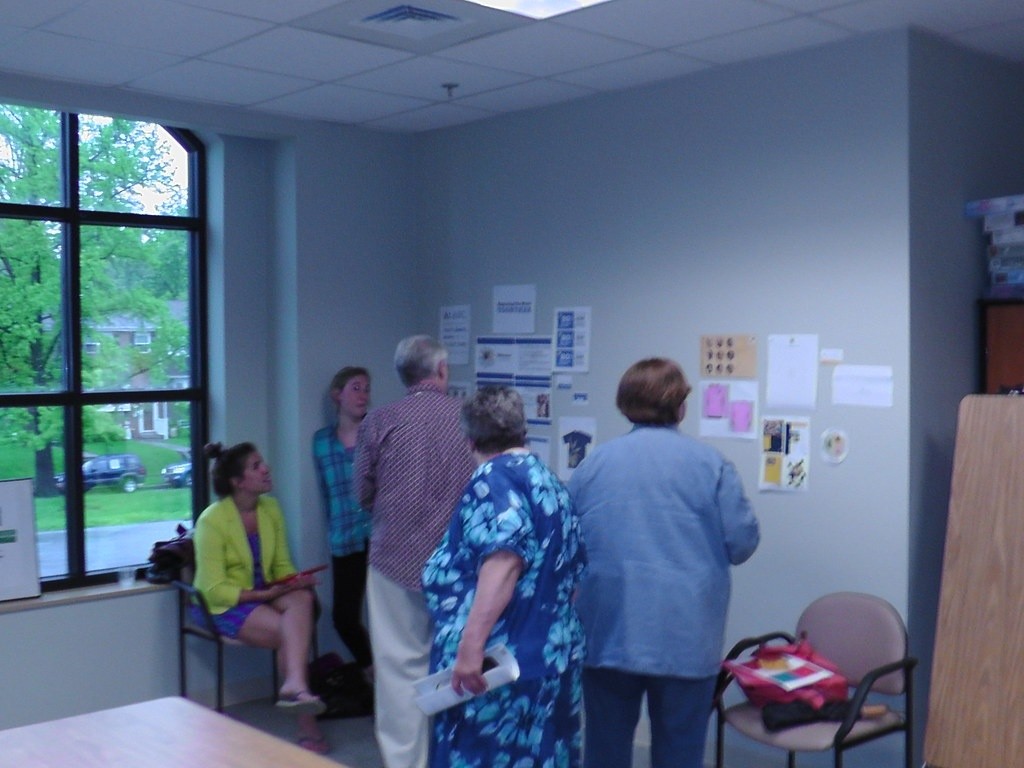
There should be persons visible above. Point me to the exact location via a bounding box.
[194,335,589,768]
[566,355,760,768]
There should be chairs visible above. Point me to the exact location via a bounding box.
[171,534,321,714]
[707,596,914,768]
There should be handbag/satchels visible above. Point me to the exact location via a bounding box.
[712,631,848,709]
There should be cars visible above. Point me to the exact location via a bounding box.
[161,463,193,488]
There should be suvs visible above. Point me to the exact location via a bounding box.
[54,453,148,497]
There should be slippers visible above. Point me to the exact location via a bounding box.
[276,692,326,715]
[300,733,330,753]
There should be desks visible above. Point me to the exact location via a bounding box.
[0,695,354,768]
